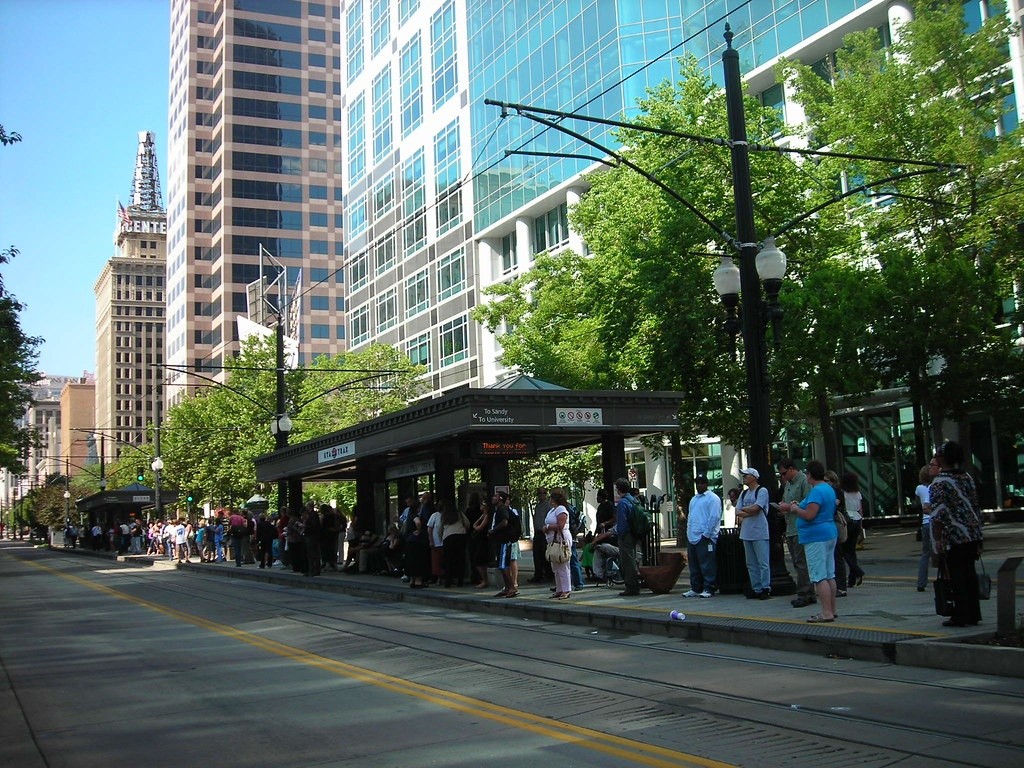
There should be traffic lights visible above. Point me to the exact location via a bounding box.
[187,491,193,504]
[137,467,144,481]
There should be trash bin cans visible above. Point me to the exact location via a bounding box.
[716,527,746,594]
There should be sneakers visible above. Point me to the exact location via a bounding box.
[700,590,716,598]
[682,590,700,597]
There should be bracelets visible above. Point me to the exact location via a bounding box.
[547,524,550,530]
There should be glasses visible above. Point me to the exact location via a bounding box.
[929,463,939,466]
[780,469,790,476]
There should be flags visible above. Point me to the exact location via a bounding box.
[117,200,129,223]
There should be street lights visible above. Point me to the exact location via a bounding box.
[151,456,164,519]
[270,411,293,512]
[64,490,71,520]
[713,227,797,597]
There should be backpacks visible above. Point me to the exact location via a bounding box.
[741,486,777,531]
[262,524,278,545]
[507,510,522,542]
[566,503,589,535]
[623,497,653,539]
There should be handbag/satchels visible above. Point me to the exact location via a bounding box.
[858,528,866,545]
[545,528,571,563]
[834,511,848,543]
[934,579,955,617]
[231,526,244,538]
[976,573,992,600]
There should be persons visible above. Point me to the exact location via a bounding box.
[64,516,195,564]
[779,460,838,623]
[735,468,771,600]
[682,473,722,598]
[196,501,362,576]
[778,460,865,608]
[526,487,583,600]
[590,477,640,596]
[915,441,985,627]
[363,490,522,597]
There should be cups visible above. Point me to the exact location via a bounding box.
[669,610,685,620]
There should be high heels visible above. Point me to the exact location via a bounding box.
[847,570,865,588]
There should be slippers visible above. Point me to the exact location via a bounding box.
[807,613,838,623]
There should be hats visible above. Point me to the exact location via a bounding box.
[693,474,708,482]
[739,467,760,479]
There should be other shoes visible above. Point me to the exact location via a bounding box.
[550,591,570,600]
[836,590,847,597]
[409,582,428,589]
[917,586,925,592]
[620,590,640,596]
[791,592,818,608]
[550,587,556,591]
[942,617,965,628]
[495,589,520,599]
[575,587,583,591]
[747,590,771,600]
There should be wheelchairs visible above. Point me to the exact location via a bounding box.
[582,545,641,588]
